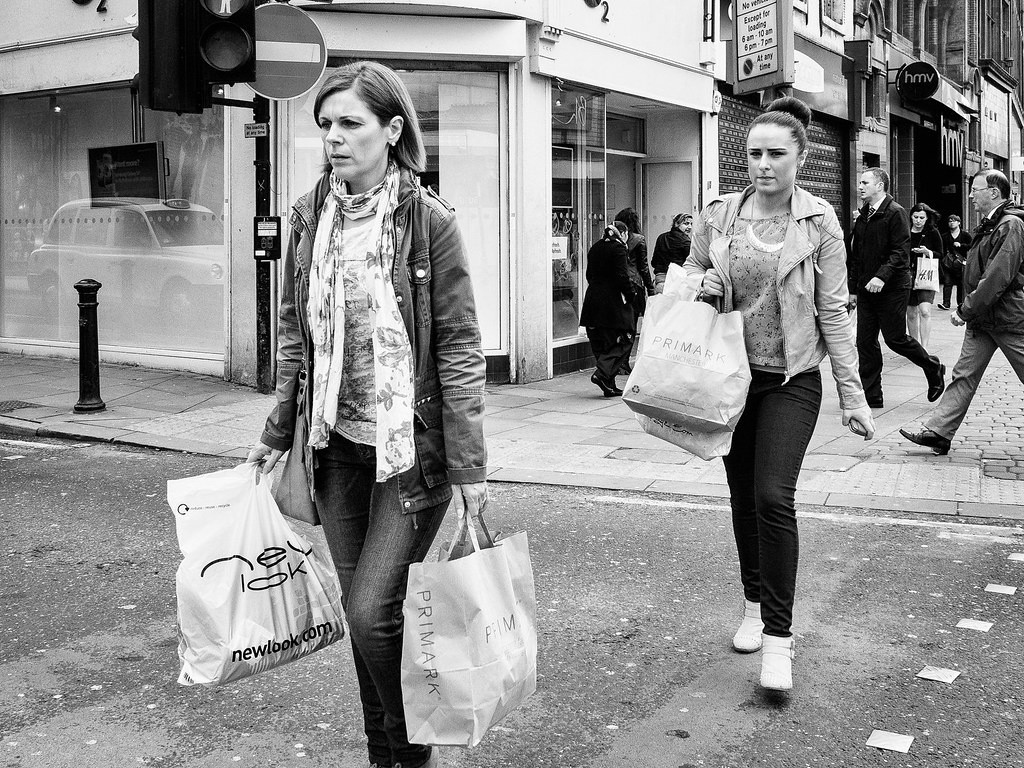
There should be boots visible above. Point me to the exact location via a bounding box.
[760,633,795,692]
[732,598,766,654]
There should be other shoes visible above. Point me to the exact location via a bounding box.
[937,303,950,310]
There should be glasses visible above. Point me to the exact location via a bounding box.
[970,186,993,191]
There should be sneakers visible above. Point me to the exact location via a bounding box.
[590,370,626,397]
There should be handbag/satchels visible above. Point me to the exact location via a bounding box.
[164,459,344,688]
[940,249,968,275]
[912,250,941,293]
[397,496,539,751]
[619,265,753,462]
[272,399,323,527]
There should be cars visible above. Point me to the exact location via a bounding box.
[26,195,224,315]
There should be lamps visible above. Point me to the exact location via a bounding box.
[1001,57,1013,68]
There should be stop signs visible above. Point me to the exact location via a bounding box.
[245,2,329,103]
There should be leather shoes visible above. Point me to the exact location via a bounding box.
[867,395,885,407]
[899,428,951,456]
[927,363,946,402]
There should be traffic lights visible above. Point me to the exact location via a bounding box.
[188,0,256,85]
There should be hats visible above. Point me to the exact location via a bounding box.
[670,213,693,229]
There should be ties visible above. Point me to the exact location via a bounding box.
[864,207,875,224]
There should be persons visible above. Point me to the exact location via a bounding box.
[245,59,488,768]
[907,202,943,352]
[651,213,693,294]
[937,214,972,310]
[844,168,946,410]
[613,207,654,375]
[899,168,1024,454]
[679,97,876,692]
[579,222,637,396]
